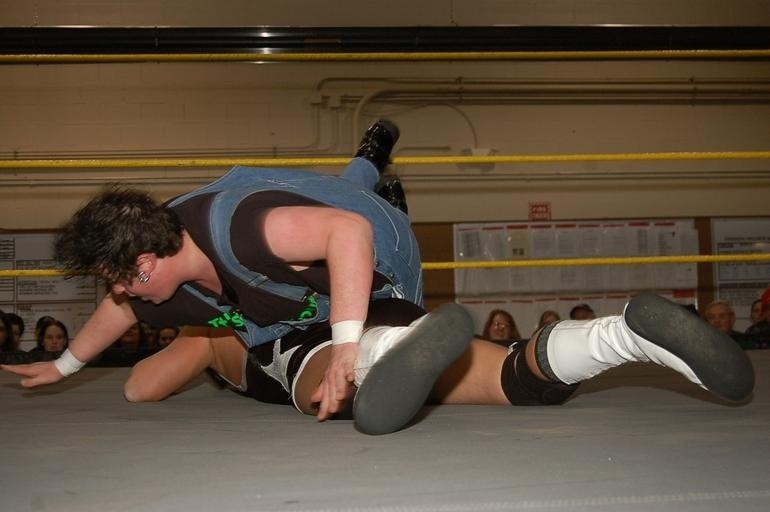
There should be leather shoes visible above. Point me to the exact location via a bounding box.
[372,175,409,215]
[354,117,400,172]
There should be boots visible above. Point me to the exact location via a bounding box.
[349,303,476,436]
[534,291,757,404]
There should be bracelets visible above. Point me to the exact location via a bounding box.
[54,349,85,378]
[324,321,364,346]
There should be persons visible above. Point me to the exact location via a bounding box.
[1,118,425,422]
[0,309,181,367]
[482,289,770,352]
[125,297,756,435]
